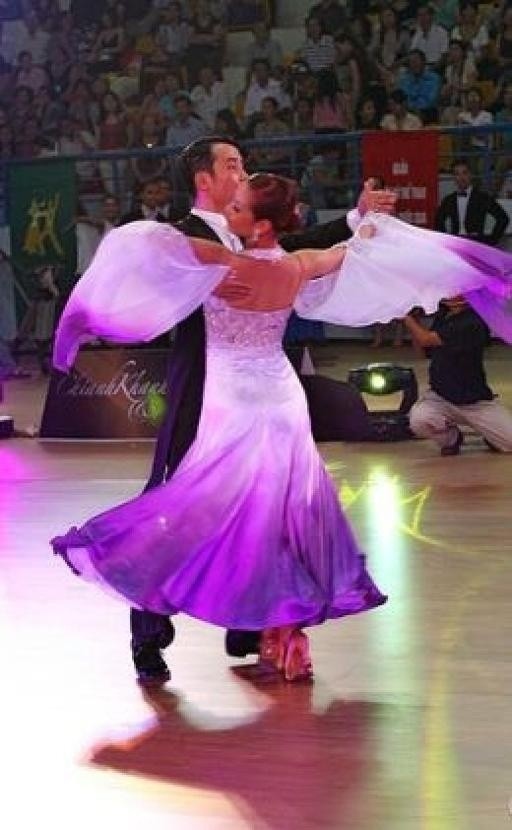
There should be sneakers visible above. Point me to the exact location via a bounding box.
[439,433,468,456]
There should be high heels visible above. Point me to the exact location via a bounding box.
[275,628,316,684]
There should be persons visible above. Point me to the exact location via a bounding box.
[128,135,399,688]
[0,1,512,380]
[403,289,511,456]
[46,173,511,683]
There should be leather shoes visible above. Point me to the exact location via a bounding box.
[225,626,266,658]
[129,640,173,685]
